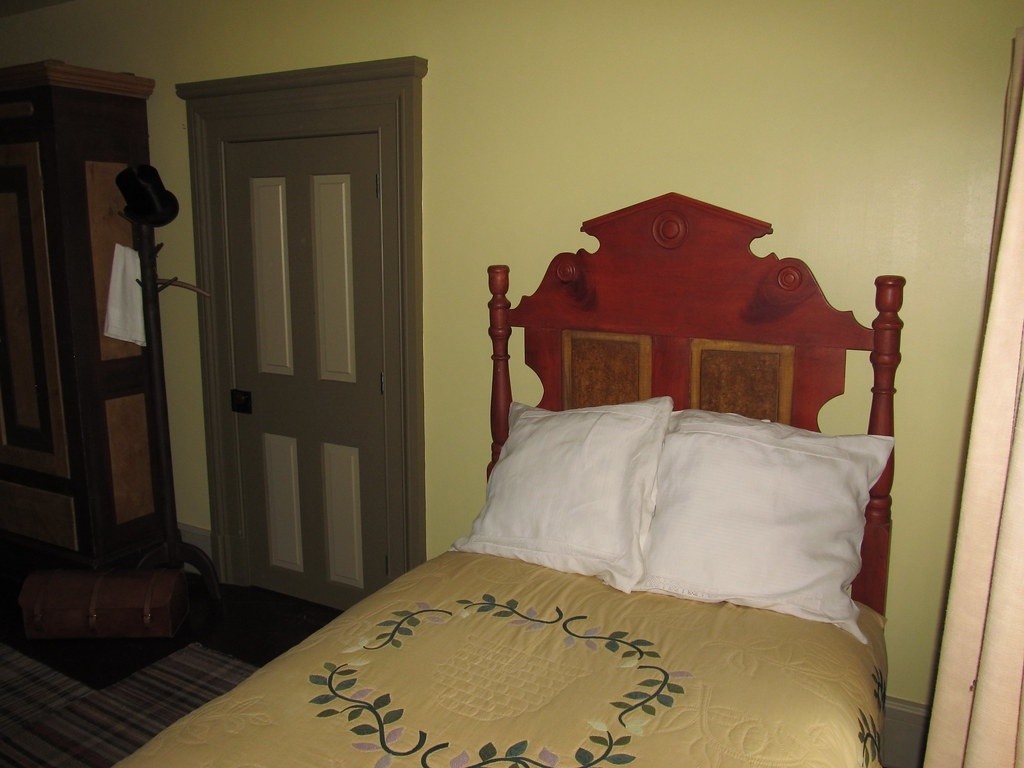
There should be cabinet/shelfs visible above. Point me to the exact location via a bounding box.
[0,58,182,578]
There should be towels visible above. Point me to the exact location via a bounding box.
[104,243,146,346]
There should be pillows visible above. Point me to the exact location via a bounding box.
[628,408,894,648]
[447,396,675,593]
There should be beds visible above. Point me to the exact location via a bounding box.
[112,192,906,768]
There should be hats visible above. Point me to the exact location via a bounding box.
[113,163,180,228]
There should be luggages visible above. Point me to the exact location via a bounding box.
[18,568,190,640]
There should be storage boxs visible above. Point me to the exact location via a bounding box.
[17,570,192,641]
